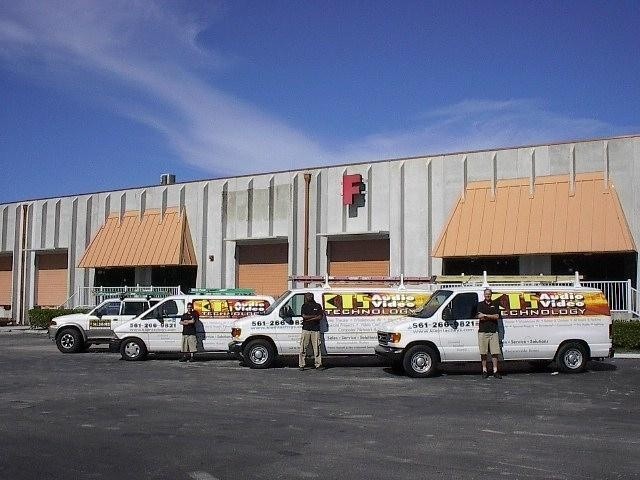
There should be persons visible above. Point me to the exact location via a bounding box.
[475,288,504,379]
[179,302,199,362]
[298,293,326,371]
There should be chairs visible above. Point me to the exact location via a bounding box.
[454,293,476,318]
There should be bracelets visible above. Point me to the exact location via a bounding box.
[484,314,487,319]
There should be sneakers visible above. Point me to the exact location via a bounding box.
[300,367,304,370]
[179,357,188,362]
[482,371,488,378]
[493,371,502,379]
[317,366,325,371]
[190,356,197,362]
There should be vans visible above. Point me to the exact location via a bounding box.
[113,294,274,361]
[377,285,615,371]
[230,285,427,364]
[46,299,162,352]
